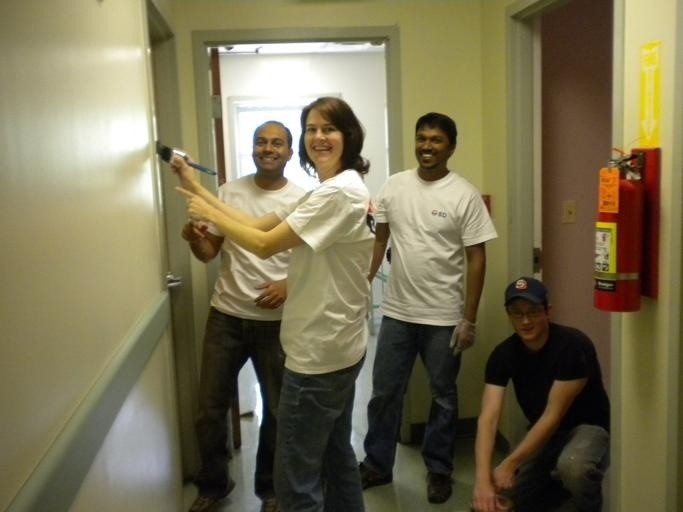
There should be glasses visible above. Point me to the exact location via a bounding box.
[509,307,540,319]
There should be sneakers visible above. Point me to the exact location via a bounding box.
[187,478,235,512]
[358,461,391,489]
[426,468,452,504]
[261,495,279,512]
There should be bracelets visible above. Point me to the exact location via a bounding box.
[460,316,479,328]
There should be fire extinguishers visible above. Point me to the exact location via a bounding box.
[593,151,646,314]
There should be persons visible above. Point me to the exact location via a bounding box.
[356,107,500,504]
[468,274,615,511]
[163,94,376,511]
[177,117,309,511]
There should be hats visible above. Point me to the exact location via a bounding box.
[505,276,547,305]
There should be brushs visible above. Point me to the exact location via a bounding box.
[156,140,217,176]
